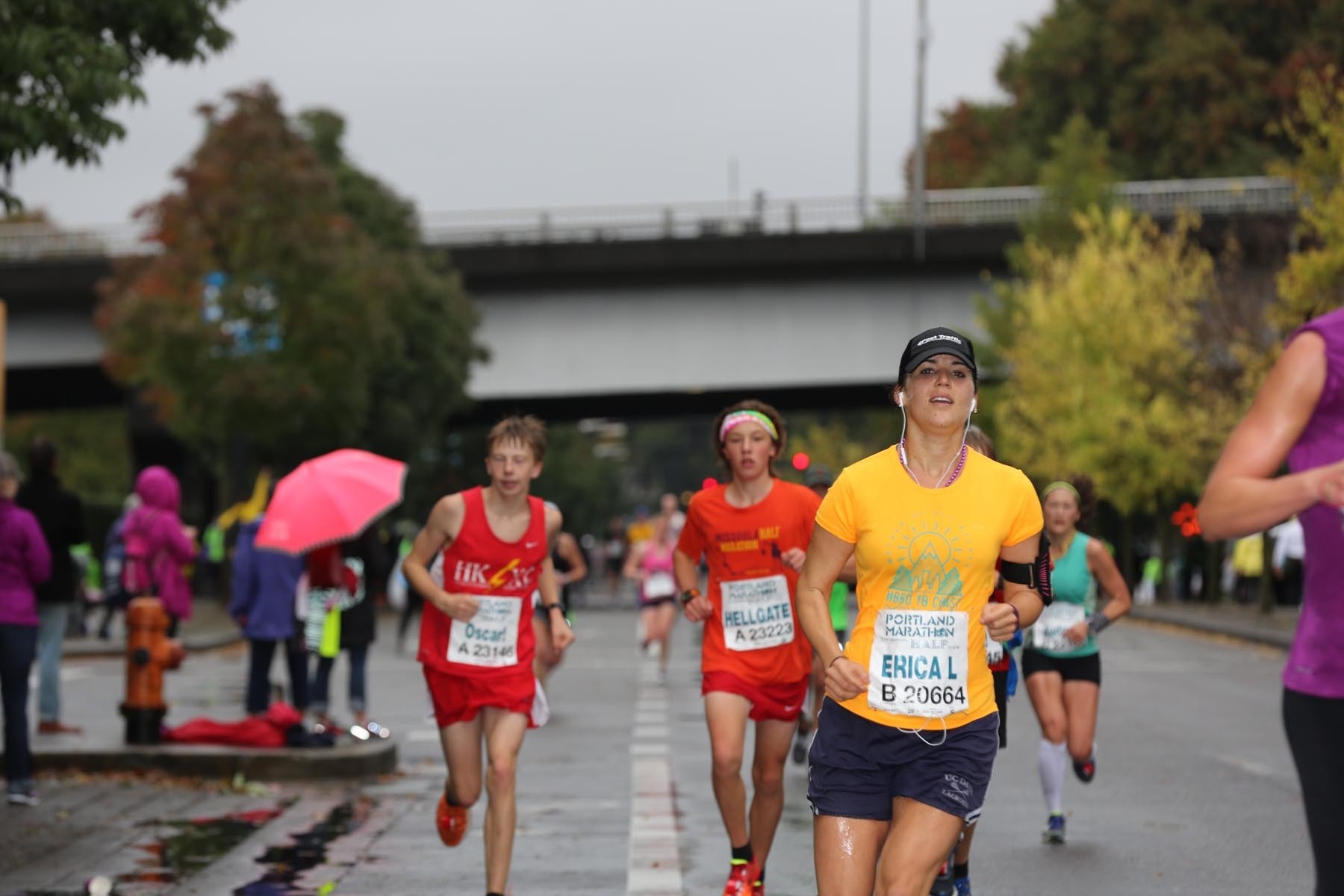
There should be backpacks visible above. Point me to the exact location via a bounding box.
[118,505,164,595]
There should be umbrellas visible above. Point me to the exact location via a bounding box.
[254,450,407,553]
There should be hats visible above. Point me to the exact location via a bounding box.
[898,326,977,389]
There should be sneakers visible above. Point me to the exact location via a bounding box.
[752,871,766,896]
[792,718,813,764]
[956,875,971,896]
[722,852,760,896]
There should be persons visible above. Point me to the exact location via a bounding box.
[798,327,1053,896]
[929,428,1025,896]
[66,466,1304,764]
[14,437,85,736]
[1023,483,1132,844]
[1195,308,1344,896]
[674,400,819,896]
[0,449,51,812]
[402,414,575,896]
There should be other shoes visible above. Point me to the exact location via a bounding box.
[37,722,81,735]
[436,779,467,846]
[1073,756,1095,782]
[353,710,370,729]
[1045,811,1065,844]
[6,785,41,808]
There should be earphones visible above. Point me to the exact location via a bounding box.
[970,398,976,411]
[898,392,904,405]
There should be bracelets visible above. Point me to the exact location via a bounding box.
[829,655,846,667]
[1085,610,1110,634]
[1009,604,1019,633]
[680,588,701,606]
[546,602,565,610]
